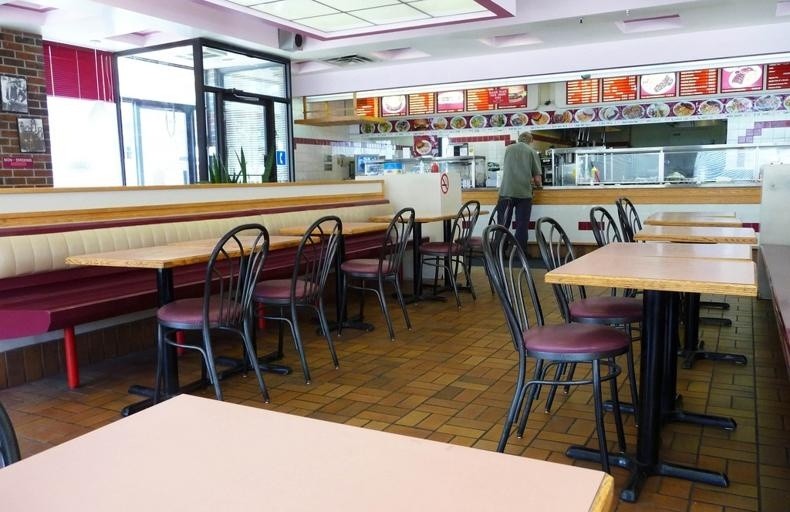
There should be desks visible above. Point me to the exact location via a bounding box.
[273,221,391,337]
[0,385,616,512]
[365,209,489,307]
[642,210,744,310]
[588,240,751,369]
[633,225,757,329]
[544,254,761,503]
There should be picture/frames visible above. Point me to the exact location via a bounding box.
[0,73,47,154]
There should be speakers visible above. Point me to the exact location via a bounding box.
[279,29,303,51]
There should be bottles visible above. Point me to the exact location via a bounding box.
[431,162,439,173]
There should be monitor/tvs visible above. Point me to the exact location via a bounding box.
[356,154,380,176]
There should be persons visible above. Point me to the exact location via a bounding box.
[488,131,543,260]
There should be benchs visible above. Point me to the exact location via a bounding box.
[0,198,429,390]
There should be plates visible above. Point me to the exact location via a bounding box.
[359,94,790,136]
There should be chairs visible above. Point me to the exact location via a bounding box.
[588,196,647,342]
[534,217,636,416]
[415,198,481,310]
[154,222,274,405]
[485,224,635,473]
[216,214,343,385]
[336,207,417,341]
